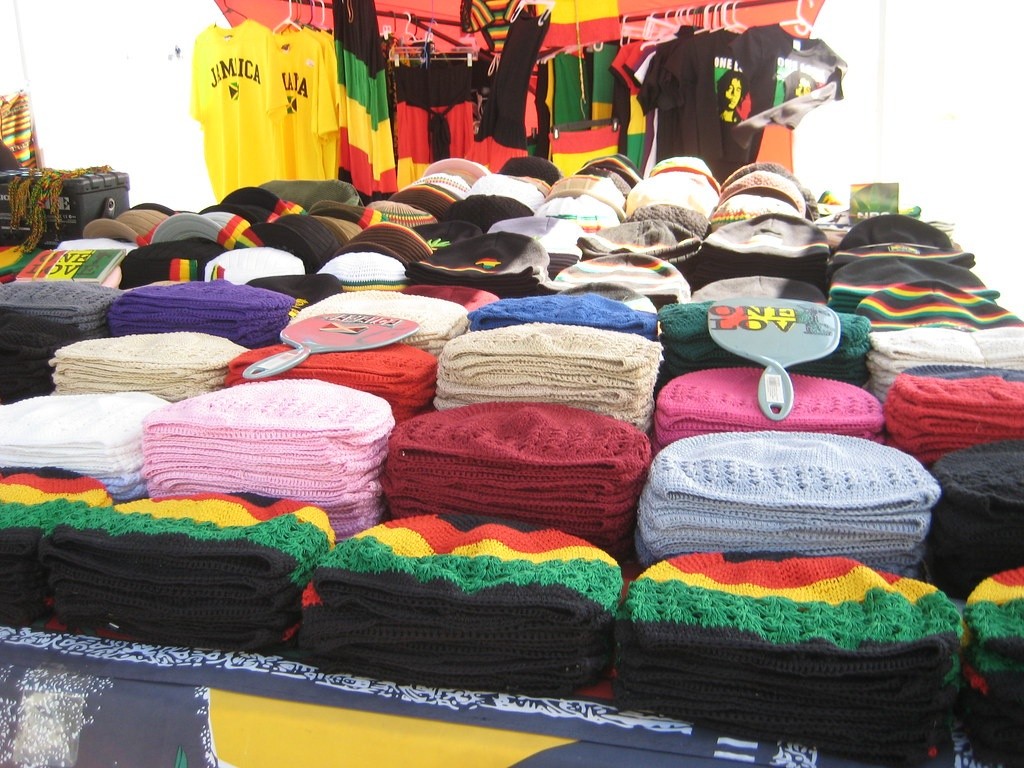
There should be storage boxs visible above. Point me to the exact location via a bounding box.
[0,169,130,249]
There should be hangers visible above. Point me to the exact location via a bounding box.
[197,0,845,70]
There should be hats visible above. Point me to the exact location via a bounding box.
[1,156,1023,763]
